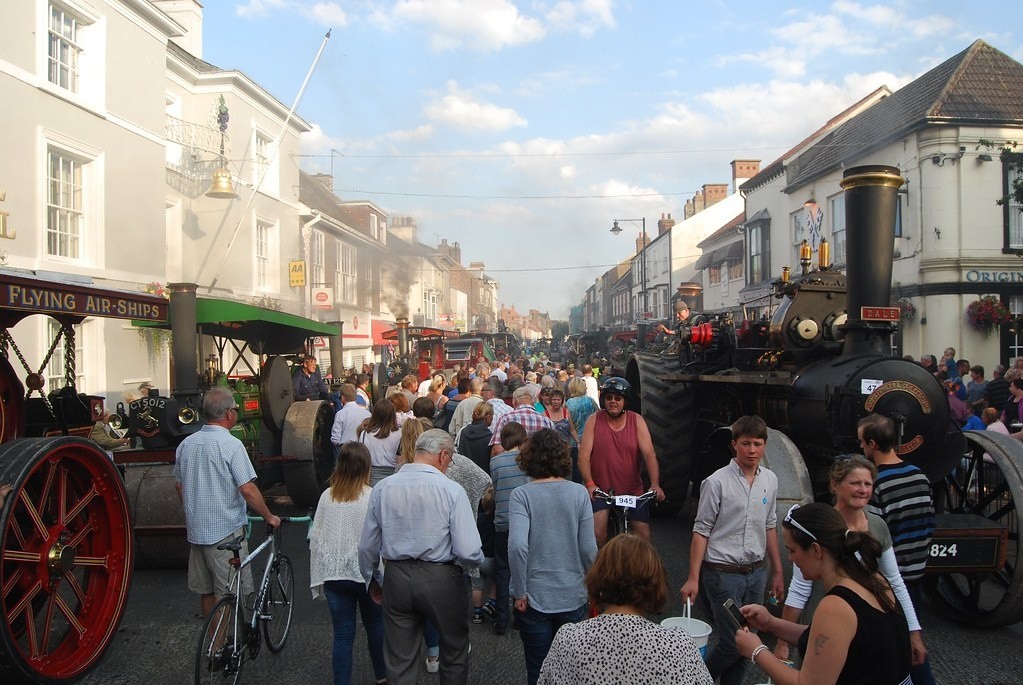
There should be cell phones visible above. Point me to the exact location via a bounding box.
[723,599,750,630]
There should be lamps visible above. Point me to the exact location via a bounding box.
[977,155,993,163]
[803,199,816,206]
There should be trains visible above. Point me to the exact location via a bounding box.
[0,260,345,685]
[369,315,496,408]
[619,161,1023,633]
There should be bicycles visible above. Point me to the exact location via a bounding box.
[192,506,315,685]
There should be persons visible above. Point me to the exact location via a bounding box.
[859,414,940,685]
[392,417,492,673]
[331,339,613,506]
[310,441,387,685]
[578,376,665,544]
[772,453,928,665]
[656,302,703,337]
[904,347,1023,512]
[88,406,129,449]
[292,355,324,402]
[680,416,786,685]
[722,501,915,685]
[490,421,535,638]
[358,429,485,685]
[536,532,714,685]
[507,428,599,685]
[171,387,281,672]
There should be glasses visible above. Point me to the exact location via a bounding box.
[440,451,455,467]
[543,392,549,397]
[605,396,623,401]
[228,407,238,414]
[784,504,823,544]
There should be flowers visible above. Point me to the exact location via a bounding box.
[968,295,1012,338]
[889,296,915,324]
[136,280,177,365]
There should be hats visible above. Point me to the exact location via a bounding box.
[673,301,687,315]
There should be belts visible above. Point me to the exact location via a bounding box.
[408,558,456,566]
[703,561,761,575]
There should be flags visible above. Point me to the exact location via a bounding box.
[388,343,397,361]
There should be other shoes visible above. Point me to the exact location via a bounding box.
[472,612,483,624]
[425,658,439,673]
[969,486,986,494]
[208,656,226,671]
[483,598,497,615]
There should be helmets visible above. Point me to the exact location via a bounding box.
[600,377,633,409]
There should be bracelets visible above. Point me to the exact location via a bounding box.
[751,645,769,666]
[584,479,594,487]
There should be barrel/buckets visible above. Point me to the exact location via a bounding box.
[660,596,712,662]
